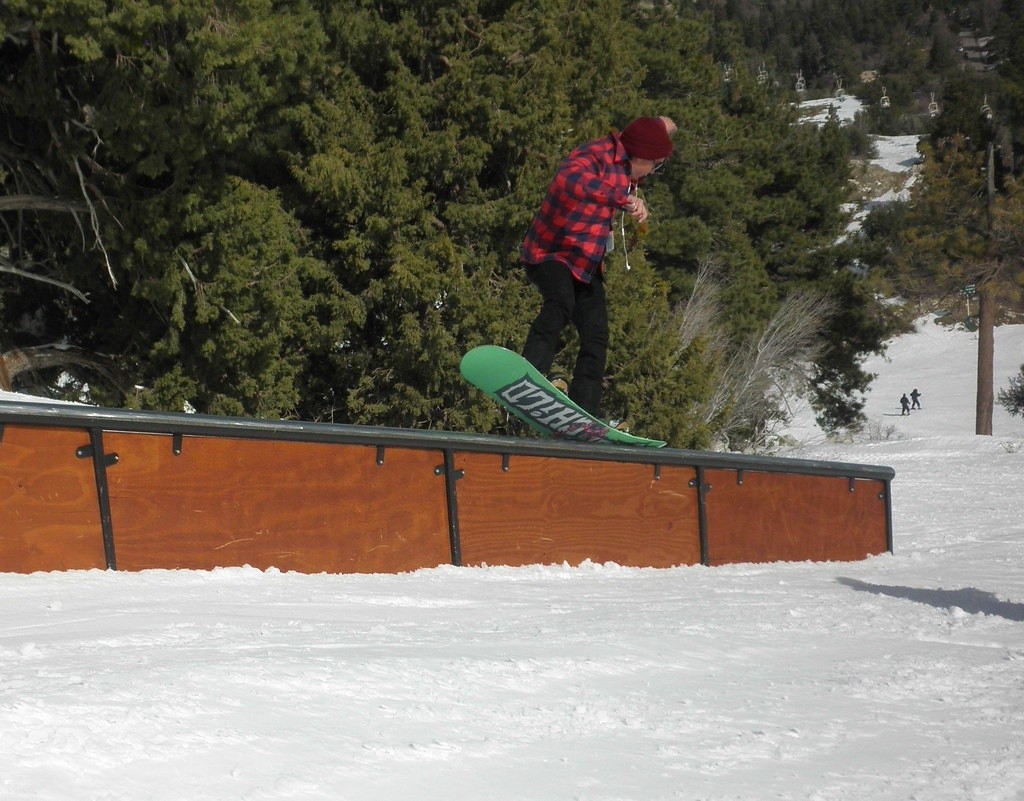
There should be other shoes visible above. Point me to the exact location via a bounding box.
[608,419,628,431]
[550,378,568,394]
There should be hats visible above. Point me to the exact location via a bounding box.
[620,116,673,160]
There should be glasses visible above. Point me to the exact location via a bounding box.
[651,156,663,171]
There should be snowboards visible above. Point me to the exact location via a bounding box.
[460,345,667,448]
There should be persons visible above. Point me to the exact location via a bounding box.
[900,393,911,415]
[518,117,677,433]
[910,389,921,409]
